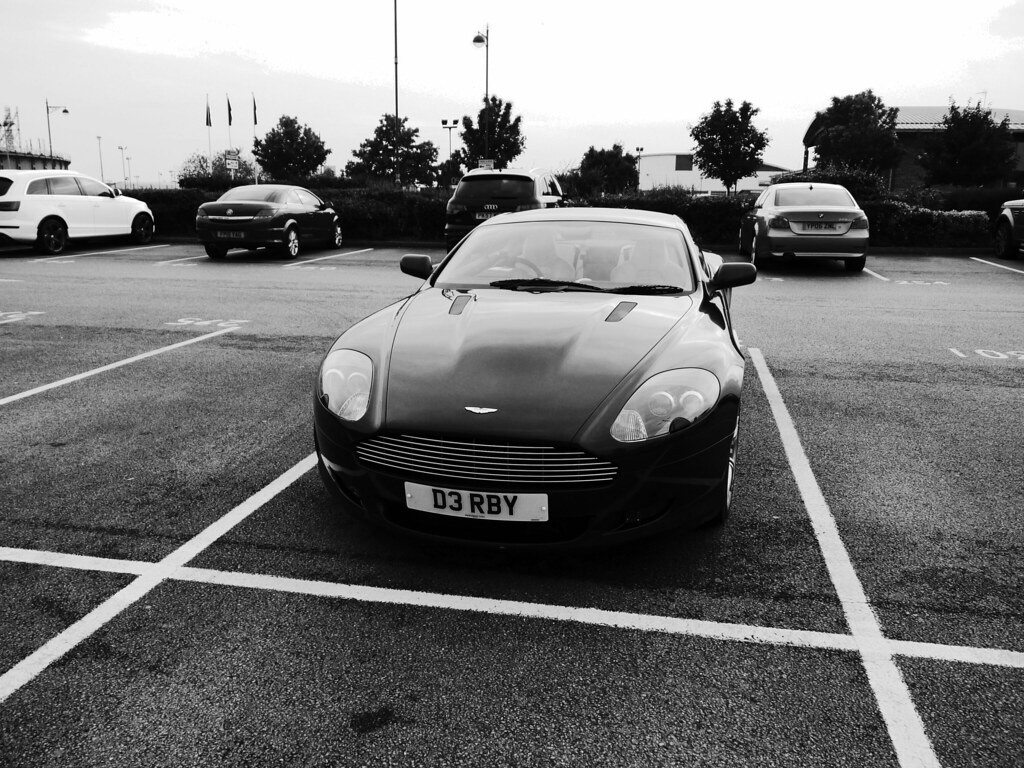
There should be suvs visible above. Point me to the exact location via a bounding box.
[0,168,157,254]
[444,167,569,255]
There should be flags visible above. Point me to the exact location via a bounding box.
[253,98,258,125]
[227,100,232,126]
[205,103,212,127]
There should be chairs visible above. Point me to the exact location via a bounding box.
[608,243,678,286]
[515,231,574,282]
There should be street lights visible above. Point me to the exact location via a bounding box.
[441,119,459,167]
[118,146,132,189]
[97,135,103,183]
[635,147,643,197]
[46,99,69,169]
[473,22,489,159]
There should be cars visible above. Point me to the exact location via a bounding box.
[195,183,344,260]
[739,181,869,274]
[315,205,757,550]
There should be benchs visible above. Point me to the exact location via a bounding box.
[556,240,624,283]
